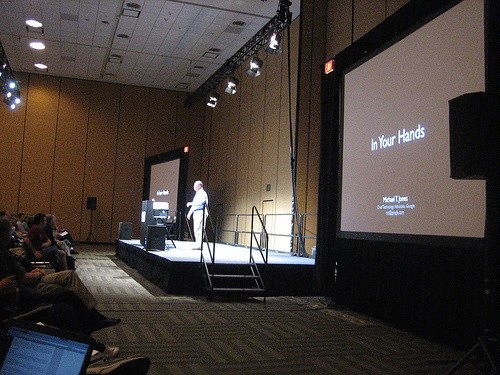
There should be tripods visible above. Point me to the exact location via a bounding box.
[83,210,97,244]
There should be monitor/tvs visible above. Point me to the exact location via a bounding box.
[0,318,96,375]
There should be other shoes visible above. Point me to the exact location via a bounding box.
[192,248,201,250]
[92,317,121,332]
[71,242,80,246]
[70,249,78,254]
[97,354,151,375]
[87,346,120,367]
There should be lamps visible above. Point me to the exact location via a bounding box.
[225,72,240,95]
[266,32,282,54]
[248,55,263,78]
[0,58,20,109]
[207,86,220,108]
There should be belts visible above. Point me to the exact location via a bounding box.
[194,209,203,211]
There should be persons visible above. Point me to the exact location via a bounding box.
[186,179,209,250]
[0,210,152,375]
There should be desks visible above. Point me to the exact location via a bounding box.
[153,215,177,248]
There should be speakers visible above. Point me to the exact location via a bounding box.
[448,91,500,180]
[118,222,131,239]
[87,197,96,209]
[147,225,166,251]
